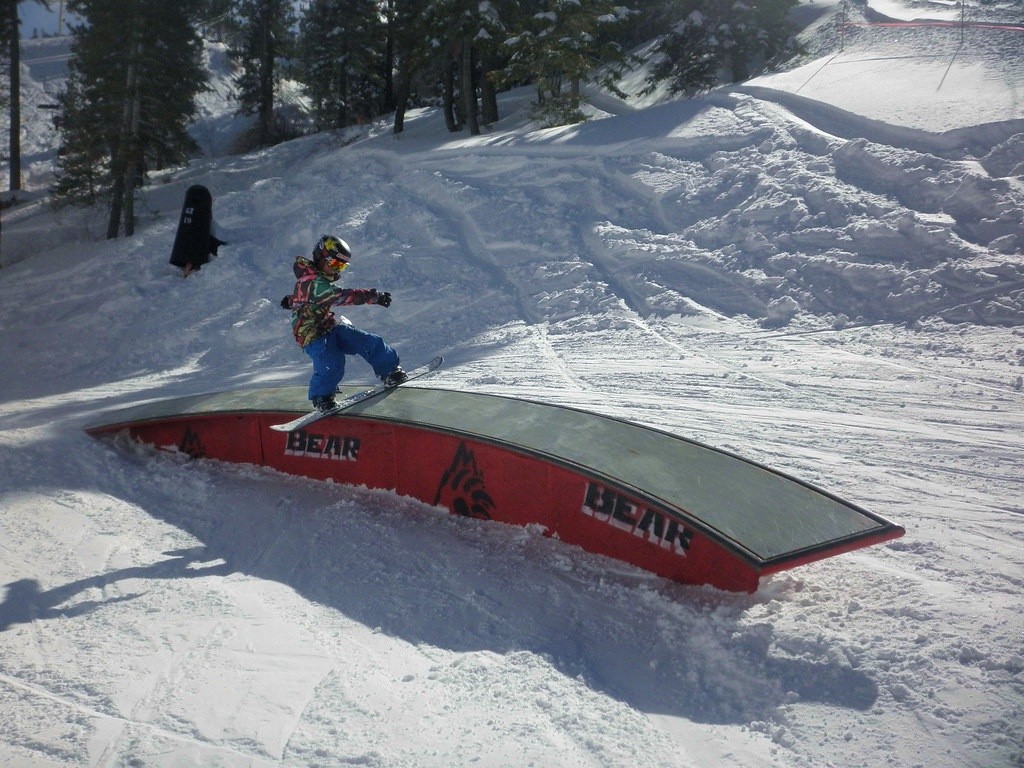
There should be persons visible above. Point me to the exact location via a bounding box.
[281,235,408,415]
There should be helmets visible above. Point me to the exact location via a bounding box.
[313,235,351,266]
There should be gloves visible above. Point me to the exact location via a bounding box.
[280,295,293,309]
[372,288,392,308]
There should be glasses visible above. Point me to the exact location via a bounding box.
[328,259,350,273]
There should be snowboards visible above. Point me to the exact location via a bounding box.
[268,356,443,431]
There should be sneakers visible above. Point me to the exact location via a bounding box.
[384,366,407,388]
[312,394,340,416]
